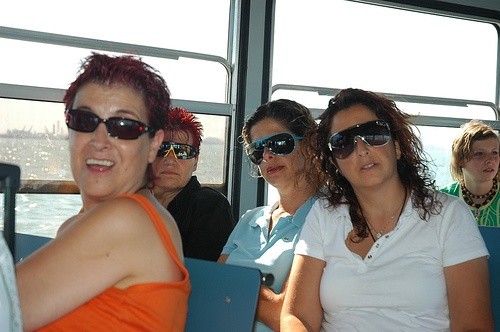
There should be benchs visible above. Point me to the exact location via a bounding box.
[12,233,261,332]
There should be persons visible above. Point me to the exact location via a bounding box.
[16,52,189,332]
[148,107,238,262]
[436,118,500,228]
[216,99,333,332]
[280,88,497,331]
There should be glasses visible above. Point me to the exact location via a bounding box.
[157,141,197,159]
[328,119,390,159]
[245,131,305,165]
[66,108,151,140]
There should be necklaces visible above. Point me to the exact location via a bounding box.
[461,177,497,221]
[359,185,407,242]
[361,201,403,238]
